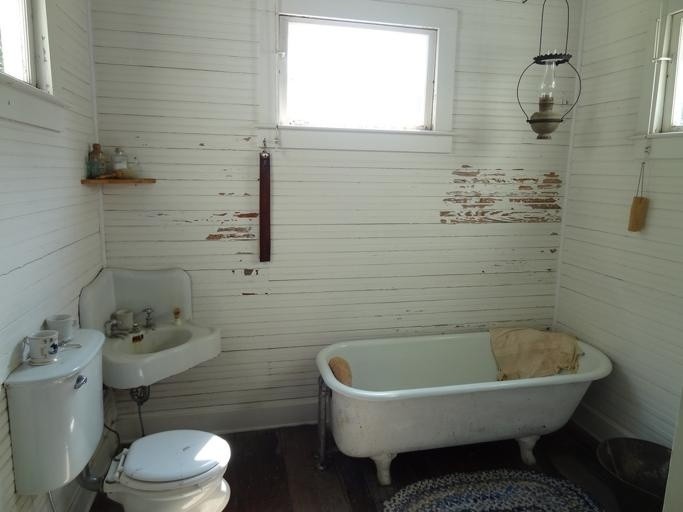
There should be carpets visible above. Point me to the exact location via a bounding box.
[379,467,603,510]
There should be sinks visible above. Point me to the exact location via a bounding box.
[115,328,192,355]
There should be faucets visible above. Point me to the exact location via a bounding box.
[104,319,128,338]
[144,308,157,331]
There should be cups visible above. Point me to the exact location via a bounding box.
[115,309,134,334]
[45,313,78,344]
[22,330,59,366]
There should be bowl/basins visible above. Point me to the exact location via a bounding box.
[593,434,673,512]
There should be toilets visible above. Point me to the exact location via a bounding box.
[4,329,231,512]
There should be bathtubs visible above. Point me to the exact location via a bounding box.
[316,331,613,487]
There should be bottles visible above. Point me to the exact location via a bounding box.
[110,147,127,172]
[86,142,106,178]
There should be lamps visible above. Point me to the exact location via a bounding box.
[515,0,584,141]
